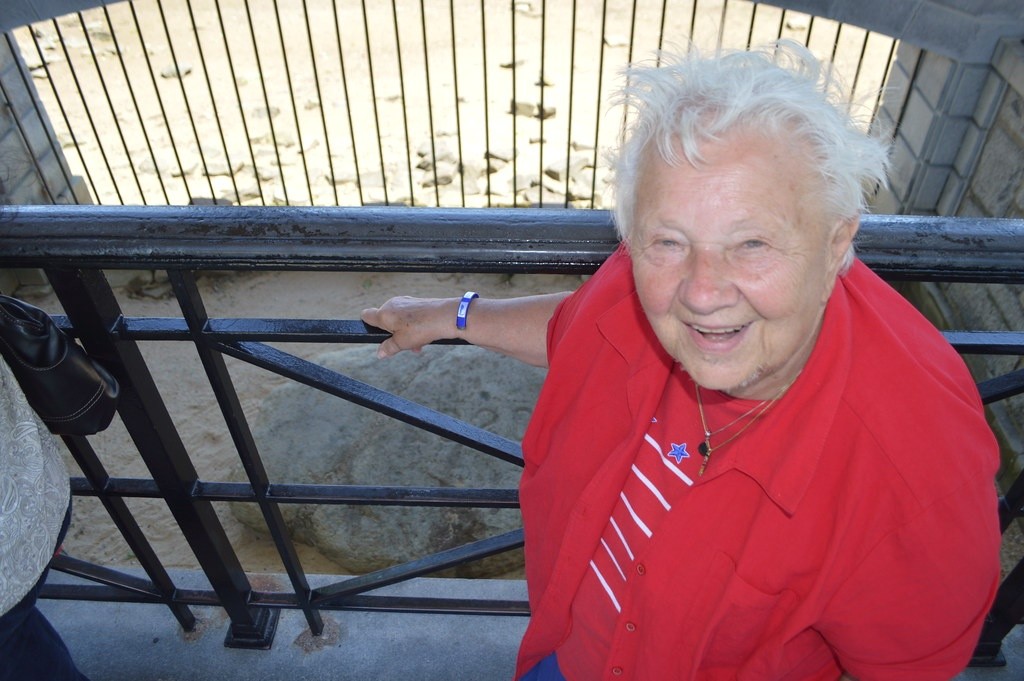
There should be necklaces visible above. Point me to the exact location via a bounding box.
[692,366,802,478]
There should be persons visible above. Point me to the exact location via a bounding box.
[356,46,1003,681]
[0,293,93,680]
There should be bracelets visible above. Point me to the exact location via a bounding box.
[455,291,479,331]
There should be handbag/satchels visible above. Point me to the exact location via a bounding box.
[0,295,120,435]
